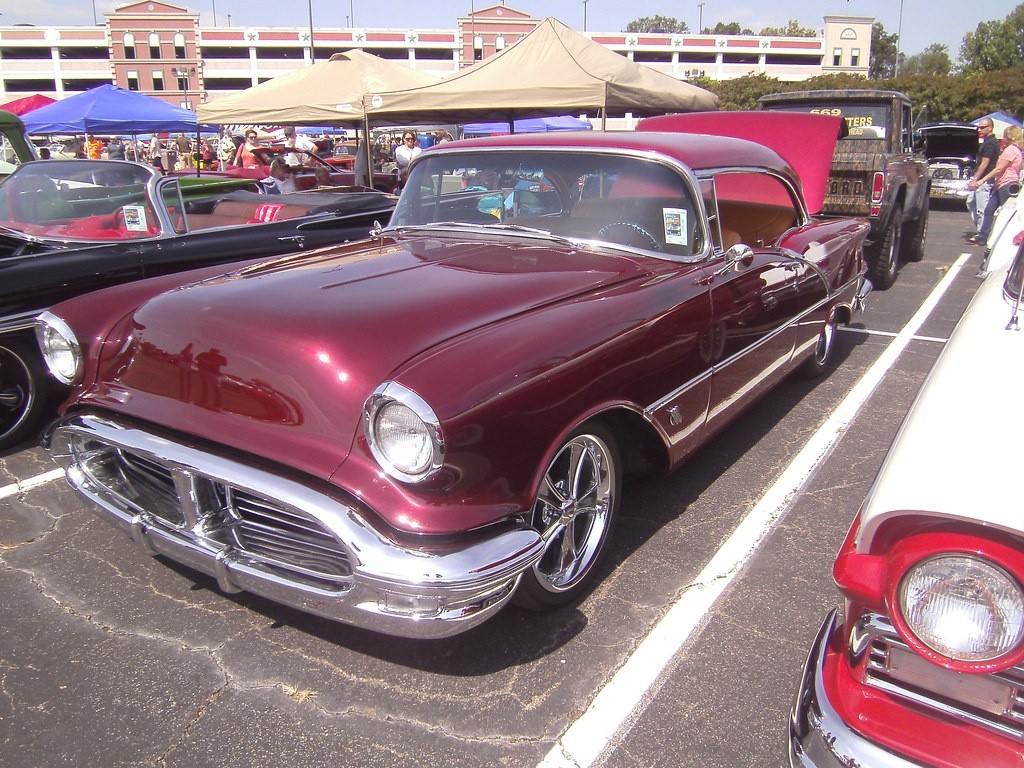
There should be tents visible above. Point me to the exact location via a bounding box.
[0,17,719,177]
[970,112,1022,143]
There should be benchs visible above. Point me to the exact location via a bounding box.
[500,195,798,256]
[210,202,309,224]
[147,212,267,233]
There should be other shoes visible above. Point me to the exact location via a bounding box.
[966,233,985,246]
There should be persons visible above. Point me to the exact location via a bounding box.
[976,126,1024,246]
[40,149,50,159]
[106,137,125,160]
[324,133,329,138]
[284,126,318,166]
[149,134,165,175]
[216,129,236,172]
[124,146,134,161]
[170,133,194,169]
[965,118,1000,241]
[436,128,451,144]
[200,136,216,170]
[395,130,422,191]
[233,130,264,168]
[339,136,344,143]
[83,132,103,159]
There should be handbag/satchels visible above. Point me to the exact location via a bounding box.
[237,157,243,168]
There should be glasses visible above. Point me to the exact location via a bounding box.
[248,136,257,139]
[404,137,414,141]
[977,125,989,130]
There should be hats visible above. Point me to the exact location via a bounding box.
[284,126,295,134]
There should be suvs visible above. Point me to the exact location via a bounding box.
[751,89,932,289]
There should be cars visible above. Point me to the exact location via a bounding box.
[915,120,983,206]
[40,112,876,643]
[332,144,360,162]
[0,160,501,451]
[168,142,397,191]
[783,172,1024,768]
